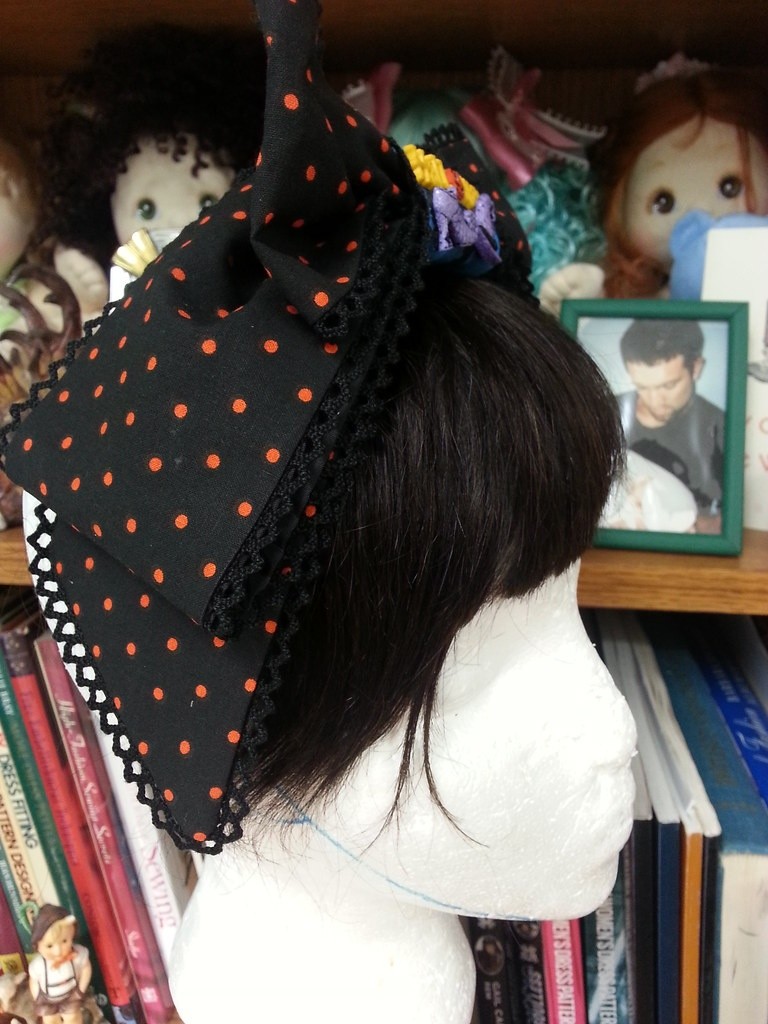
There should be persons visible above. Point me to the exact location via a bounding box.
[162,261,639,1023]
[26,902,93,1023]
[609,319,726,536]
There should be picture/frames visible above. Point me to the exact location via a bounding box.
[559,297,749,556]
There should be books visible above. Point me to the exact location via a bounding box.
[0,594,768,1023]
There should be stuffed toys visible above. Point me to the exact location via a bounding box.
[0,21,766,535]
